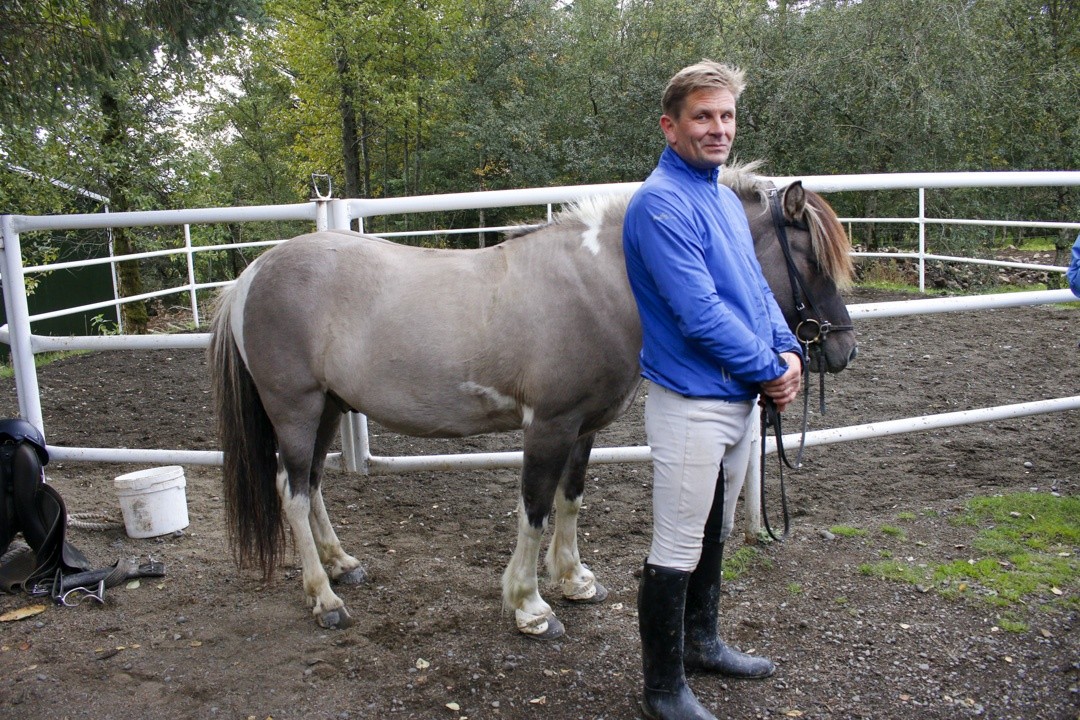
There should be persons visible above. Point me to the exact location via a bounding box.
[625,57,804,720]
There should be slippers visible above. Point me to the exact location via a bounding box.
[683,542,776,679]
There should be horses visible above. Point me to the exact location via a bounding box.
[200,146,859,643]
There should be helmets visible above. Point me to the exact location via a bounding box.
[0,420,50,466]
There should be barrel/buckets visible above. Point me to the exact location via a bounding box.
[114,465,189,538]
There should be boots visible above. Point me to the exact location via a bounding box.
[637,557,721,720]
